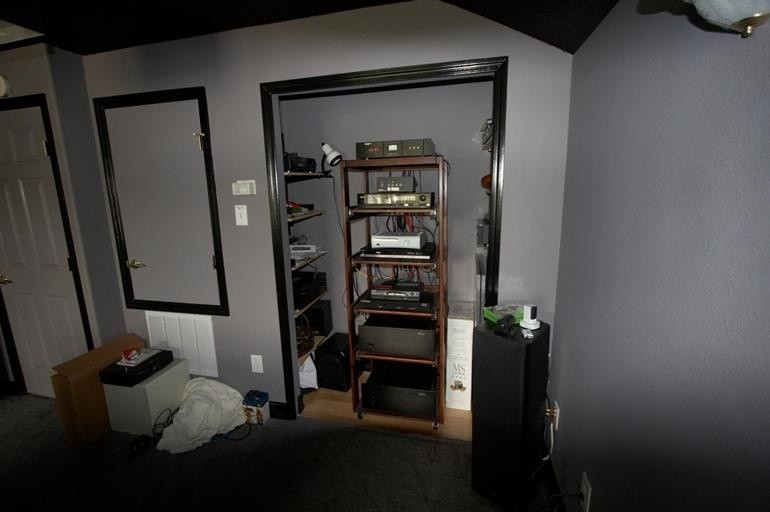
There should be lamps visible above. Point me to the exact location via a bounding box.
[692,0,770,38]
[319,141,343,179]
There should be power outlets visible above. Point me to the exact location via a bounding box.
[548,400,559,431]
[251,355,264,373]
[577,471,592,512]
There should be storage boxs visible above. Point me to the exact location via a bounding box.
[445,300,476,411]
[49,333,189,438]
[315,331,359,394]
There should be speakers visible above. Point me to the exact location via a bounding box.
[470,315,551,510]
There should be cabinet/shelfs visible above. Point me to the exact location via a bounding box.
[340,155,449,429]
[471,319,551,480]
[282,172,336,398]
